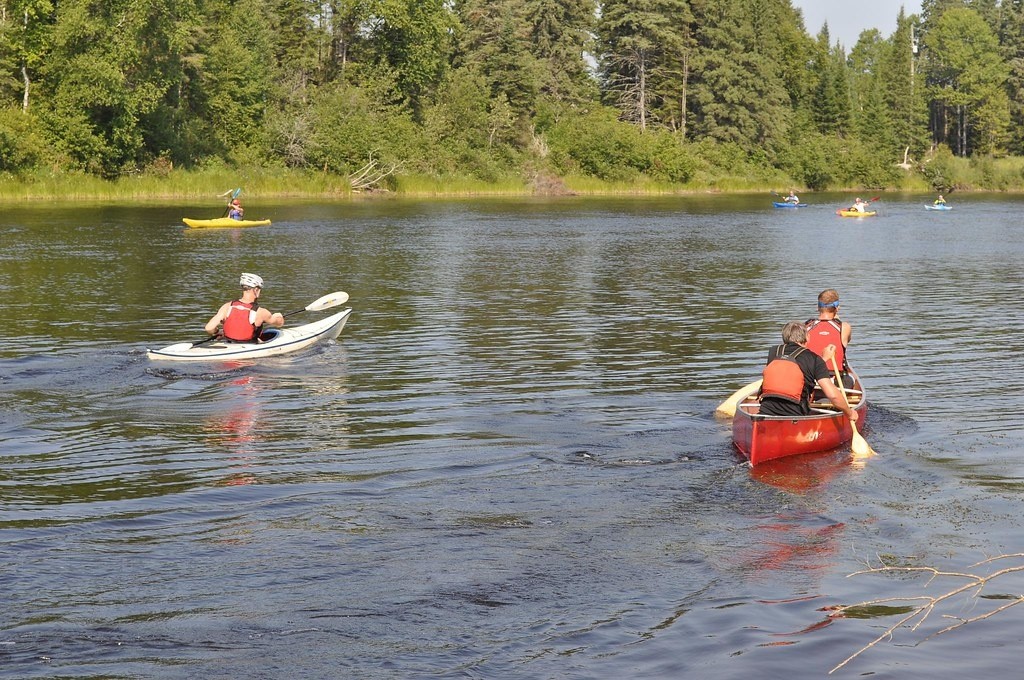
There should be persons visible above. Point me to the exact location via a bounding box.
[933,194,946,206]
[203,272,284,340]
[851,197,869,213]
[803,288,854,401]
[227,199,244,221]
[759,322,859,422]
[783,191,799,205]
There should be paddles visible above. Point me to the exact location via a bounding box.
[830,352,879,455]
[867,196,881,204]
[770,190,786,199]
[943,187,956,199]
[158,291,349,354]
[221,187,241,218]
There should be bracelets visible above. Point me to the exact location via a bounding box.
[213,328,219,334]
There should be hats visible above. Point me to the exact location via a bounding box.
[233,199,240,205]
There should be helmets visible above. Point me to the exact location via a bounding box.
[240,272,264,289]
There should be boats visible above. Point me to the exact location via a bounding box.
[733,360,867,467]
[183,217,272,228]
[772,201,807,208]
[924,205,953,211]
[146,308,352,360]
[840,210,877,217]
